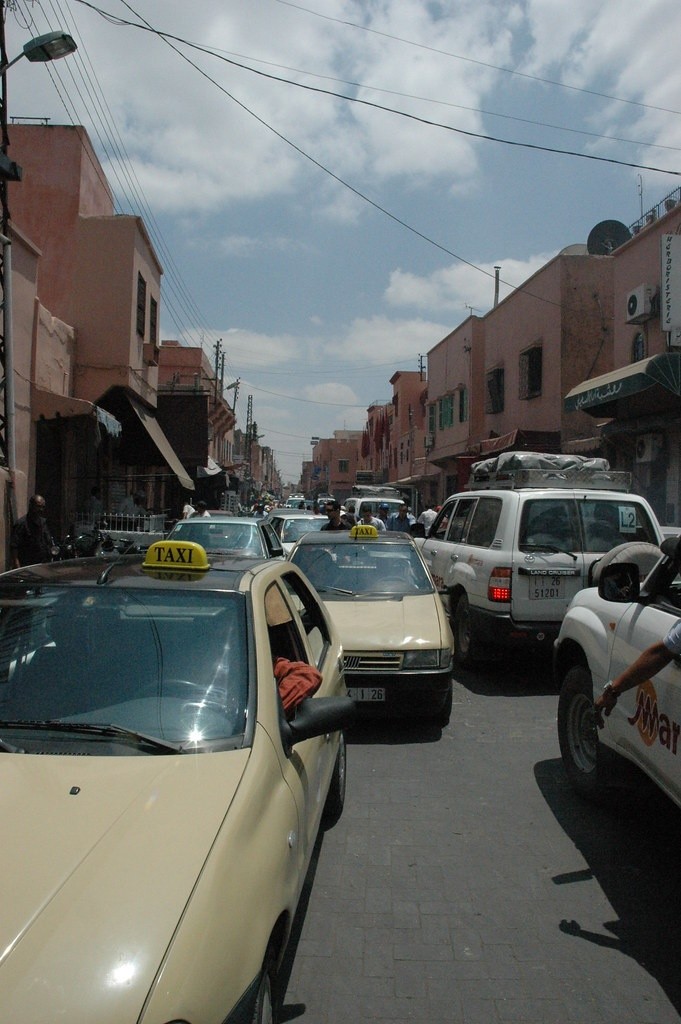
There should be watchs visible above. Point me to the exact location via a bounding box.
[603,680,621,699]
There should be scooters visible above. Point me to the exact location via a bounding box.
[46,528,146,563]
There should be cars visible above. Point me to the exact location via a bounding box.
[163,513,288,567]
[0,539,363,1024]
[264,490,419,558]
[552,522,681,817]
[292,524,455,728]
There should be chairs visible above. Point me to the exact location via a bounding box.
[300,549,347,590]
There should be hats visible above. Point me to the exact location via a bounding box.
[436,506,442,512]
[257,503,264,509]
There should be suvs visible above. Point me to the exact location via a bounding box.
[407,468,673,672]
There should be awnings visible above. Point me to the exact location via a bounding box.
[31,389,122,442]
[384,474,441,489]
[480,429,563,452]
[126,392,196,491]
[564,351,681,418]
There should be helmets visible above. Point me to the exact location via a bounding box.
[380,503,389,510]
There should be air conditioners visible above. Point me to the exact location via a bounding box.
[626,283,658,323]
[143,343,160,366]
[424,435,433,447]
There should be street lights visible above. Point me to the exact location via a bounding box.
[0,27,80,569]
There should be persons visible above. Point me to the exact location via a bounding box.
[83,489,448,536]
[10,496,56,570]
[151,615,324,718]
[363,557,416,587]
[307,561,353,591]
[593,619,681,729]
[603,573,630,600]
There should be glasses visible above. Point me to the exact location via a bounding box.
[327,509,337,513]
